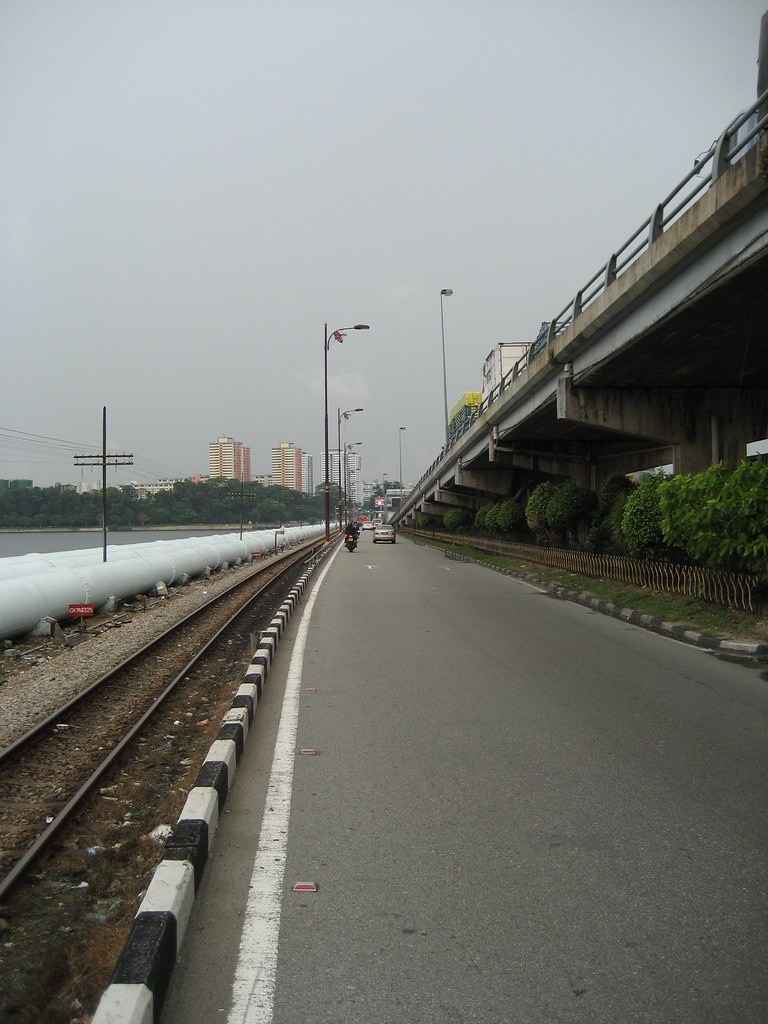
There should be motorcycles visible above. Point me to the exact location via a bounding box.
[343,523,361,553]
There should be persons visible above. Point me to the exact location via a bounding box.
[353,520,360,539]
[344,522,358,547]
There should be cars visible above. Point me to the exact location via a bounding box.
[361,521,374,531]
[372,524,397,544]
[371,518,383,529]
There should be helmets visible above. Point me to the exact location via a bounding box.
[348,522,353,527]
[353,520,357,524]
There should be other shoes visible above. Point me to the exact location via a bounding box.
[345,544,347,547]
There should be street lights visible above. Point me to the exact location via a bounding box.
[440,288,455,449]
[383,473,387,498]
[337,407,365,533]
[398,426,406,504]
[348,468,361,522]
[321,324,373,542]
[345,442,362,529]
